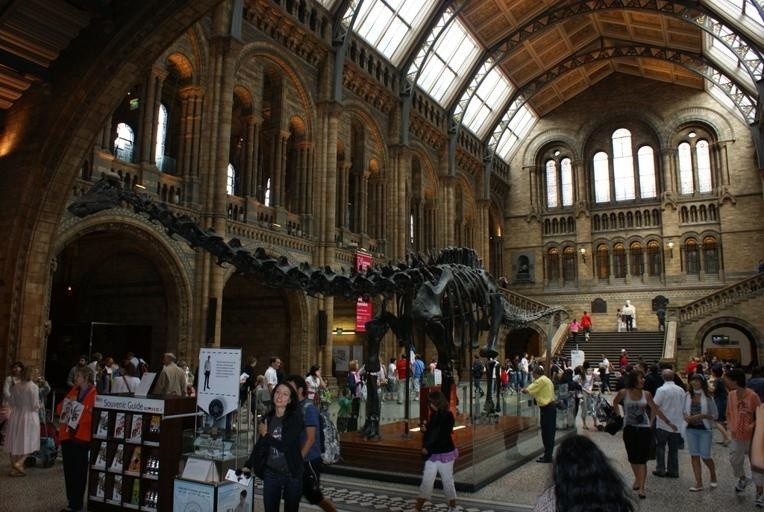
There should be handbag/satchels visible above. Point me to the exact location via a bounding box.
[25,421,61,468]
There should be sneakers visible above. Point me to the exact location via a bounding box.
[8,461,28,476]
[632,469,764,504]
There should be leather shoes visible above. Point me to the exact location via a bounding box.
[582,424,597,430]
[715,437,732,447]
[535,456,552,463]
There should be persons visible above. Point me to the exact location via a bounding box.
[657,305,665,332]
[617,303,634,332]
[499,277,508,289]
[571,312,591,344]
[1,350,764,512]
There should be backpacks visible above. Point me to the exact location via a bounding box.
[302,402,342,465]
[341,371,358,400]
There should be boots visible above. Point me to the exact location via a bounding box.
[447,499,455,508]
[403,497,426,512]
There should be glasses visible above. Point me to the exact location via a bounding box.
[235,468,251,480]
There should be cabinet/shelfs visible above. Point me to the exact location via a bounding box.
[85,395,196,512]
